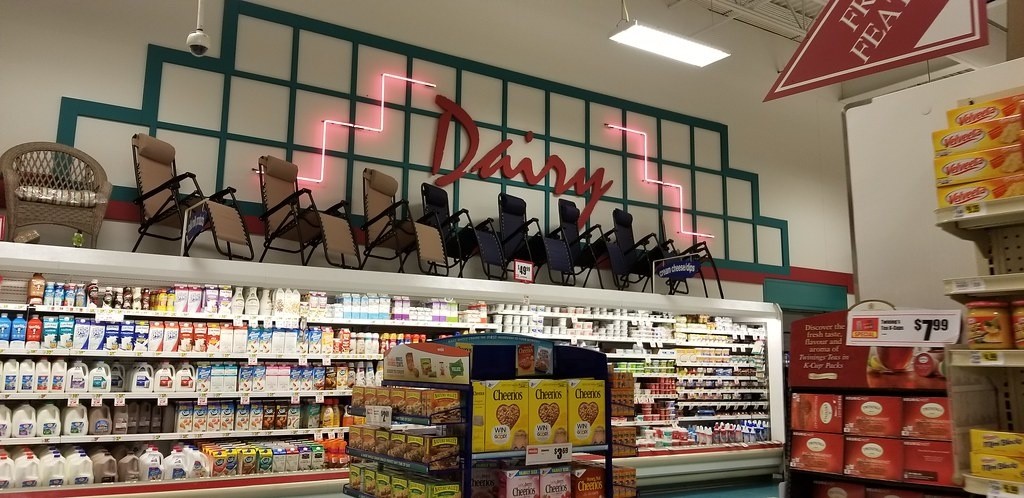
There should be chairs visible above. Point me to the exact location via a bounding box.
[0,141,113,248]
[131,132,254,261]
[258,154,362,270]
[421,182,509,280]
[497,192,576,286]
[612,207,690,295]
[359,168,450,277]
[558,198,630,290]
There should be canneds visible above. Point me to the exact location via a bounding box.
[965,299,1024,351]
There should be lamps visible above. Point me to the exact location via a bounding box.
[607,0,734,68]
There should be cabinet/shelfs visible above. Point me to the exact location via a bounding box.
[342,344,640,498]
[933,196,1024,498]
[0,241,786,498]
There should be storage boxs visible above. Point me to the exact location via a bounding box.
[787,392,1024,498]
[930,93,1024,208]
[41,283,770,498]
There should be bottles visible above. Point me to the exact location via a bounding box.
[0,402,162,438]
[118,441,210,482]
[0,444,118,489]
[686,420,770,445]
[0,358,196,393]
[350,331,460,354]
[28,272,176,312]
[232,287,273,315]
[349,361,384,386]
[320,398,354,428]
